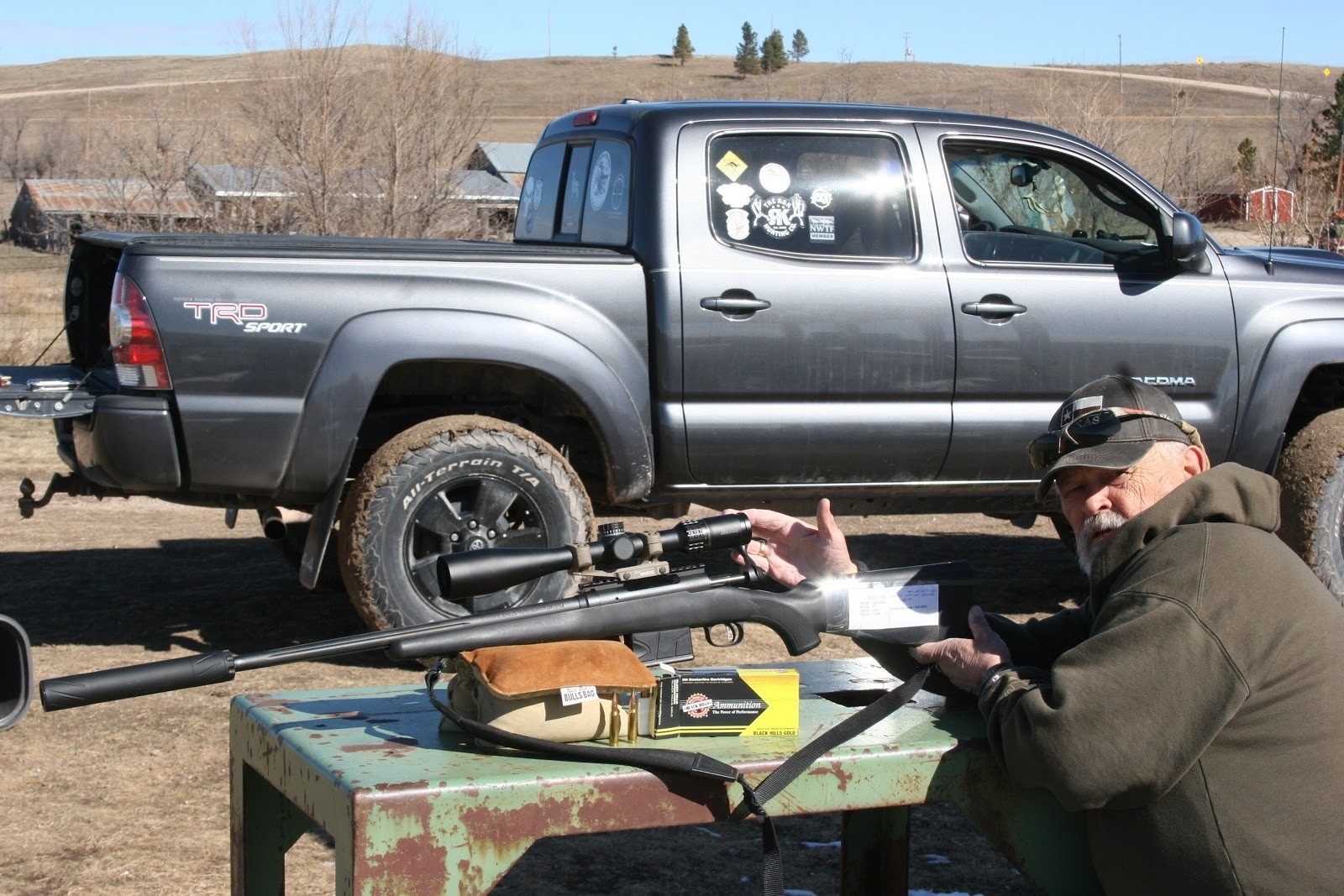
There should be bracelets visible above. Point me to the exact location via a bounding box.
[973,662,1018,707]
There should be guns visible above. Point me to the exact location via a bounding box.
[37,511,980,717]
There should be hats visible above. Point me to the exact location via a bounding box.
[1035,376,1192,503]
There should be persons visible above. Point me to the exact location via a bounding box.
[716,375,1344,896]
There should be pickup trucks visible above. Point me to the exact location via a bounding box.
[0,95,1344,658]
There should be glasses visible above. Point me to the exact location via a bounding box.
[1026,407,1182,470]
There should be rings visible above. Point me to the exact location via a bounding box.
[758,539,766,557]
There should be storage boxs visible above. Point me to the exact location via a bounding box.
[638,662,799,738]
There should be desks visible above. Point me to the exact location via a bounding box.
[231,658,959,896]
